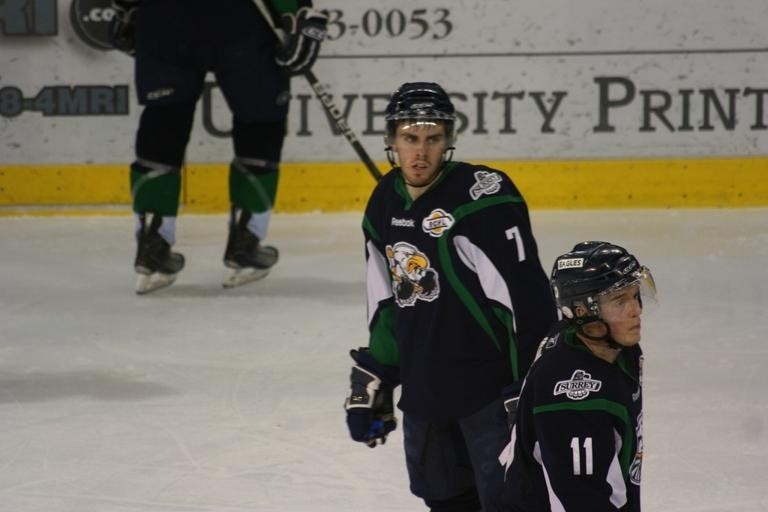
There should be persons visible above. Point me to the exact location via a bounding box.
[111,0,331,274]
[500,239,658,512]
[345,80,556,511]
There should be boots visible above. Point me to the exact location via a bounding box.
[222,204,280,270]
[132,212,187,274]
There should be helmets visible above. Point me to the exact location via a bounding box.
[549,237,660,321]
[380,81,457,152]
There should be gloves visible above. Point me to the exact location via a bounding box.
[271,5,331,77]
[107,2,138,57]
[343,346,401,448]
